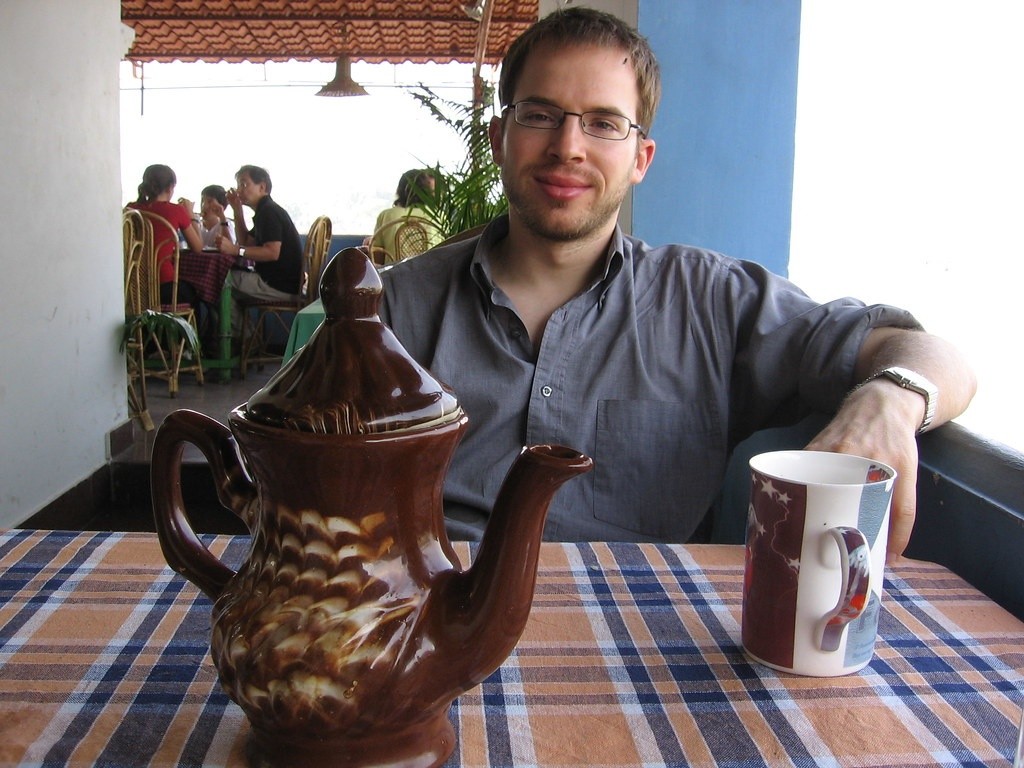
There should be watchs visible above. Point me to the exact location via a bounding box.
[220,222,229,227]
[845,366,939,434]
[237,244,246,257]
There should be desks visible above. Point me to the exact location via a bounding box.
[0,420,1024,768]
[137,240,255,383]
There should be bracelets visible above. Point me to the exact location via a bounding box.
[190,218,198,223]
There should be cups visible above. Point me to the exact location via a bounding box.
[739,449,897,677]
[225,187,239,203]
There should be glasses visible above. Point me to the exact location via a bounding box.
[499,101,649,141]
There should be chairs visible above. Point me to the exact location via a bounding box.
[239,215,332,381]
[124,206,205,399]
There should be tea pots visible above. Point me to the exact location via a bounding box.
[150,247,594,766]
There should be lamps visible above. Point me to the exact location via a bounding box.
[315,47,370,96]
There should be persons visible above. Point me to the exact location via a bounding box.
[363,168,452,265]
[376,5,978,564]
[124,164,304,356]
